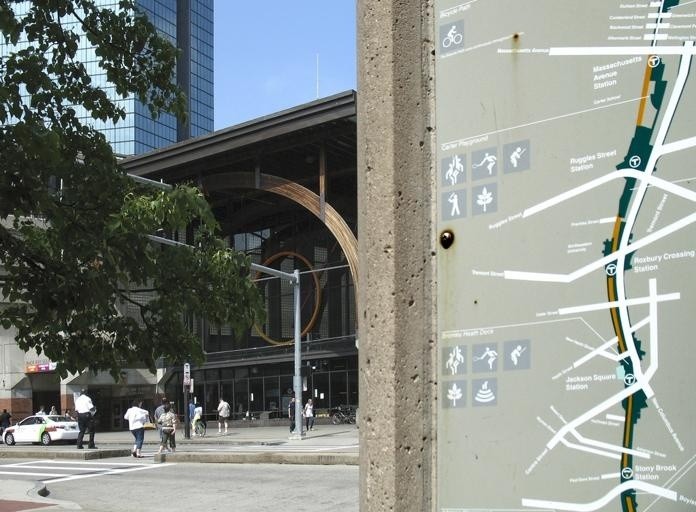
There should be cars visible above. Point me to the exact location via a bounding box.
[2,415,80,446]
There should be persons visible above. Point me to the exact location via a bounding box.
[217,397,231,436]
[123,398,149,458]
[302,398,314,431]
[287,397,295,435]
[192,403,203,436]
[158,403,177,453]
[154,397,174,452]
[188,399,196,429]
[0,389,98,449]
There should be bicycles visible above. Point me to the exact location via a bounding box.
[330,406,356,425]
[182,419,206,437]
